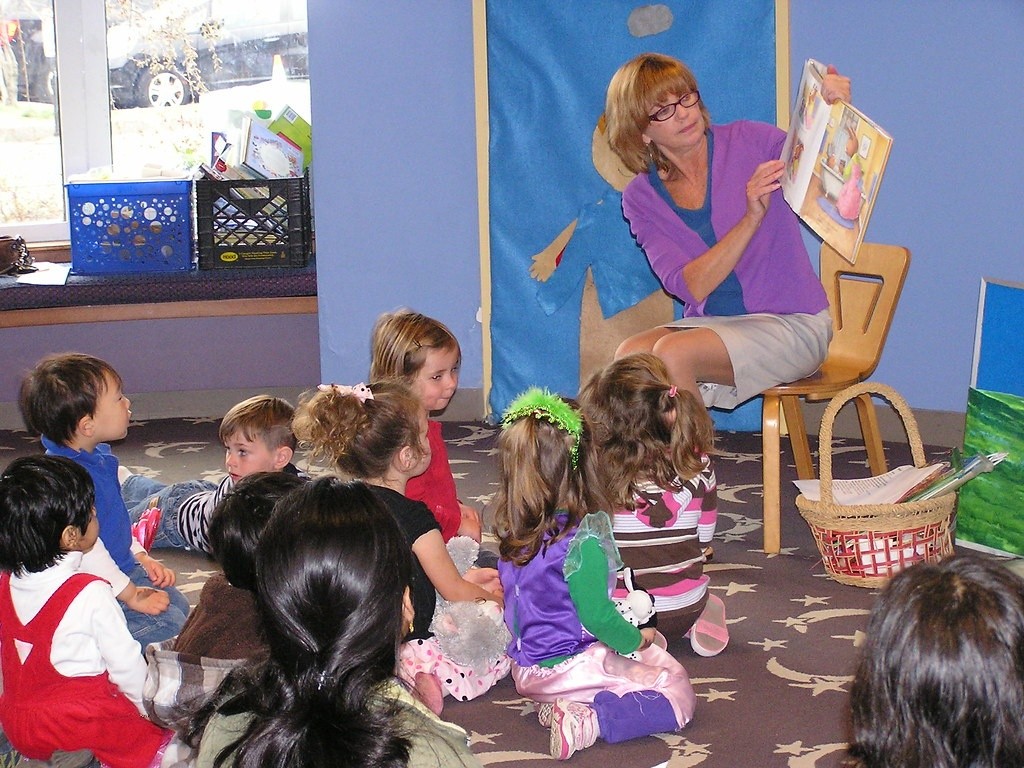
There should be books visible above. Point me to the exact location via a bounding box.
[896,446,1004,501]
[778,59,895,265]
[198,104,312,245]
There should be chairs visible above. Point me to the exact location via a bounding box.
[760,243,911,552]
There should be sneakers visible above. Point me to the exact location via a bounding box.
[537,696,554,728]
[549,695,597,760]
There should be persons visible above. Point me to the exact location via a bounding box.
[606,53,852,408]
[370,309,500,570]
[577,352,730,656]
[490,387,699,757]
[117,395,297,552]
[0,454,168,768]
[846,553,1024,768]
[196,484,484,768]
[20,353,190,654]
[143,472,305,768]
[294,381,513,715]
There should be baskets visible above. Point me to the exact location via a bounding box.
[794,379,955,589]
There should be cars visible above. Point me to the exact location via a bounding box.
[106,0,308,108]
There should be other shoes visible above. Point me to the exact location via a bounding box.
[49,749,94,767]
[131,506,161,552]
[682,594,728,657]
[413,673,444,719]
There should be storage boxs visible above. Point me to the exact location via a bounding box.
[65,179,193,275]
[195,176,311,266]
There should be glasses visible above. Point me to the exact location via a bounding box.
[646,89,701,122]
